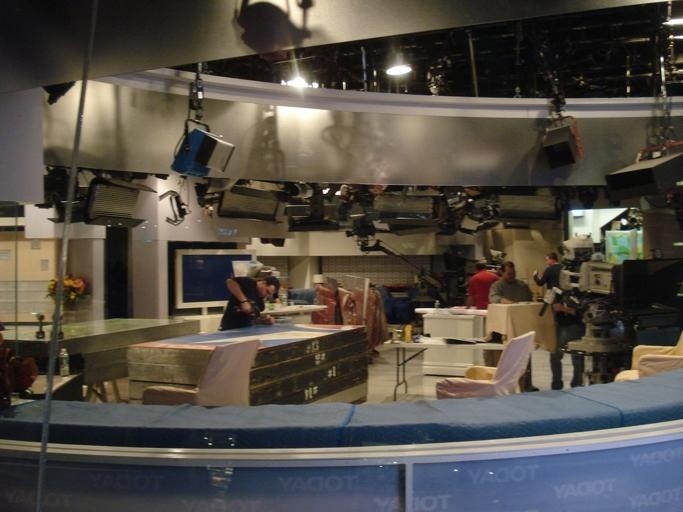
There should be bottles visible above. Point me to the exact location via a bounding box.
[391,323,421,344]
[57,348,69,377]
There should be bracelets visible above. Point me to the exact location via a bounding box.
[240,299,249,304]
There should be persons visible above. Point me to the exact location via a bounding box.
[489,262,539,392]
[551,293,587,390]
[218,276,280,330]
[466,263,503,342]
[532,252,563,290]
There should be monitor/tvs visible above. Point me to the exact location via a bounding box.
[182,255,251,302]
[620,258,683,315]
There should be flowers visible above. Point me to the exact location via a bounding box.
[47,265,91,308]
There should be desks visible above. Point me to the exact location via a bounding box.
[423,314,484,377]
[128,323,369,403]
[3,316,200,387]
[374,338,512,401]
[486,297,557,353]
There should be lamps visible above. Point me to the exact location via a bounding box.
[381,45,413,86]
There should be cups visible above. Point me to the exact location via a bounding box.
[279,293,287,304]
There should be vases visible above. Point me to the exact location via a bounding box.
[62,309,80,324]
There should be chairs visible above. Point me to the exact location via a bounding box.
[615,323,683,381]
[436,327,540,400]
